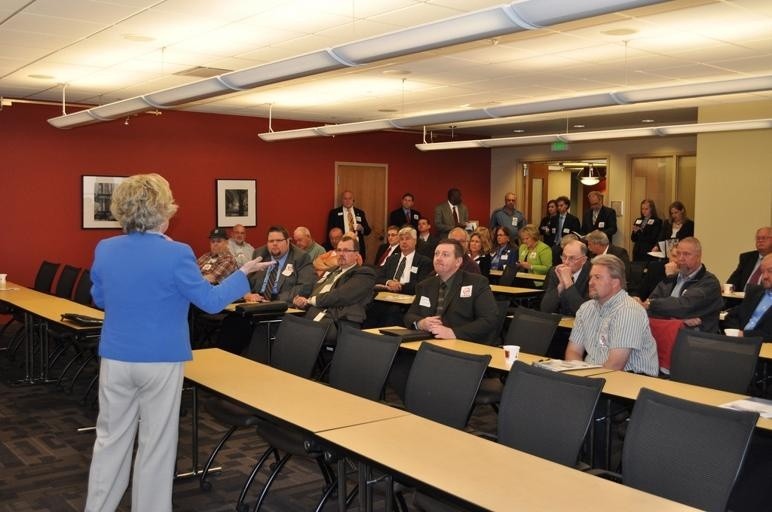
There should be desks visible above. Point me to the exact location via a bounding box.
[315,416,700,512]
[170,347,413,486]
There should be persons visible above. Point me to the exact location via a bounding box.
[566,254,659,376]
[227,224,255,269]
[293,236,377,349]
[490,191,631,318]
[720,227,771,343]
[196,229,239,286]
[81,173,279,512]
[403,239,500,345]
[631,198,721,335]
[374,190,492,295]
[244,225,317,311]
[293,191,371,277]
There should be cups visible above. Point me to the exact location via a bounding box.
[724,283,735,295]
[0,274,7,288]
[504,345,519,367]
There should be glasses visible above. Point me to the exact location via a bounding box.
[559,253,585,264]
[336,248,359,253]
[755,235,772,241]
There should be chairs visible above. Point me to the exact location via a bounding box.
[251,324,402,510]
[338,341,492,511]
[586,390,760,511]
[496,362,606,468]
[203,316,330,510]
[1,257,771,430]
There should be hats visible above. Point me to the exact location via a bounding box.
[209,228,229,240]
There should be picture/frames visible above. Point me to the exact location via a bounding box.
[81,174,130,229]
[216,178,257,228]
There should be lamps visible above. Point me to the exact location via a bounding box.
[511,0,669,27]
[257,103,329,141]
[317,119,397,135]
[143,75,247,108]
[415,126,487,151]
[577,164,602,186]
[656,119,772,135]
[613,41,772,104]
[45,86,112,129]
[390,79,494,129]
[486,92,622,117]
[477,134,566,147]
[90,96,175,119]
[559,114,663,144]
[327,4,535,67]
[220,48,361,90]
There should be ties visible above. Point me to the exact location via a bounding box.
[308,268,343,298]
[452,206,458,226]
[556,216,563,241]
[405,209,411,224]
[347,209,356,233]
[571,276,574,283]
[436,282,447,316]
[263,261,279,300]
[592,209,600,225]
[749,258,764,287]
[395,257,406,282]
